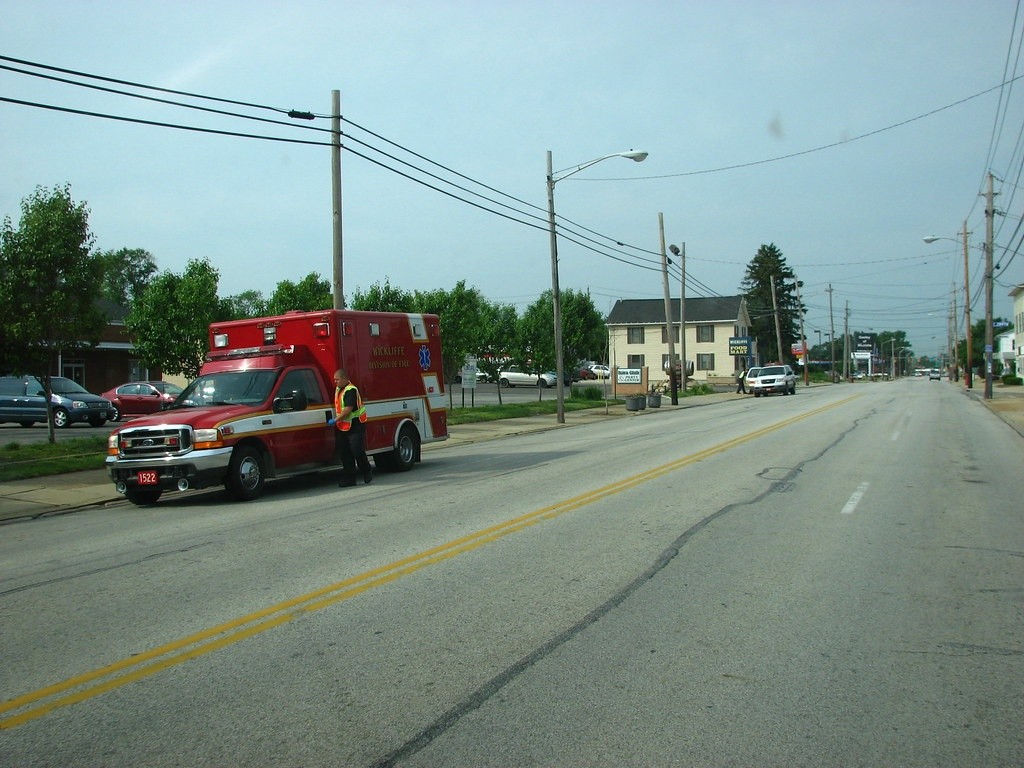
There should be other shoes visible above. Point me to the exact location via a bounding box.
[339,475,356,487]
[363,471,372,483]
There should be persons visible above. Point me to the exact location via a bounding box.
[736,369,749,394]
[328,368,372,487]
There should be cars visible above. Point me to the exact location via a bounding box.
[914,367,942,381]
[100,380,206,423]
[452,360,610,389]
[0,375,117,429]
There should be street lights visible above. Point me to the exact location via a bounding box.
[823,333,831,381]
[880,338,897,381]
[814,330,822,360]
[546,149,649,428]
[923,235,995,398]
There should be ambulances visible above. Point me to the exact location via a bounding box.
[105,308,451,507]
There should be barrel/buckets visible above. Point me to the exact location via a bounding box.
[665,360,694,376]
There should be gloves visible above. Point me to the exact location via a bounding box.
[328,419,335,425]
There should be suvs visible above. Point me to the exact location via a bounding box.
[745,364,797,397]
